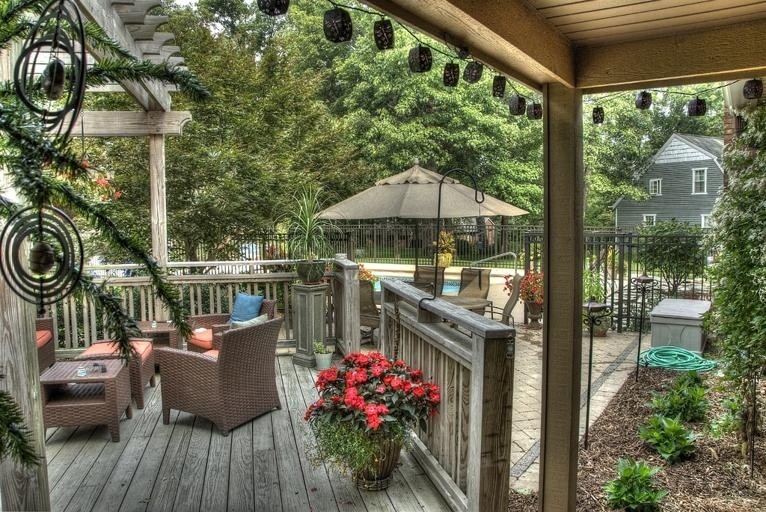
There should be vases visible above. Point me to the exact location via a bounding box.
[523,297,543,331]
[351,416,401,494]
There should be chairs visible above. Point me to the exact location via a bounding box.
[413,264,446,296]
[181,296,275,354]
[151,311,285,439]
[353,278,379,349]
[455,268,492,318]
[384,282,435,305]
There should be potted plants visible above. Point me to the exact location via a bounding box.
[431,229,456,267]
[270,177,345,286]
[311,339,333,371]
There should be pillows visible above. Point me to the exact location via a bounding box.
[226,290,264,323]
[228,312,268,330]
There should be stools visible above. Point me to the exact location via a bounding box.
[34,315,56,373]
[647,295,713,360]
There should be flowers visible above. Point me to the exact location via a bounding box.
[296,349,442,481]
[502,270,543,306]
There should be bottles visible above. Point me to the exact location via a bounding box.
[152,319,157,328]
[76,362,108,378]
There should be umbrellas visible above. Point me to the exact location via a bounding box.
[312,157,530,279]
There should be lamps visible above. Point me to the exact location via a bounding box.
[588,74,764,125]
[257,0,543,122]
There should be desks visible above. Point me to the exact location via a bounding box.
[436,295,492,317]
[76,337,156,409]
[38,360,133,444]
[127,318,183,368]
